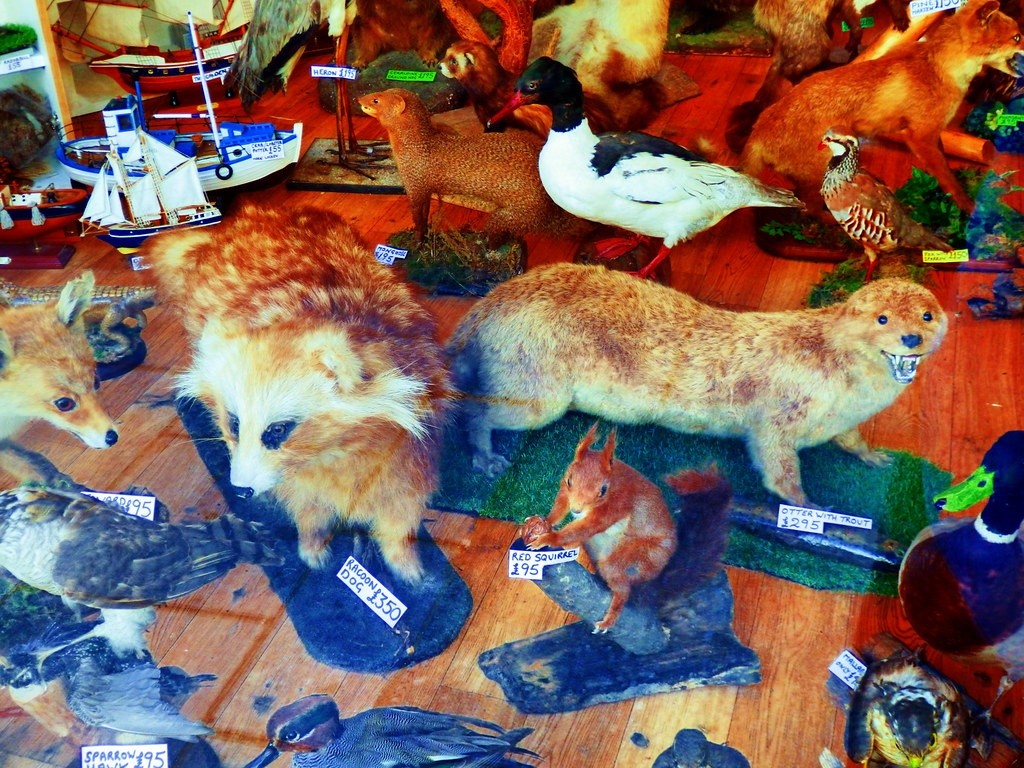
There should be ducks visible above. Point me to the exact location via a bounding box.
[896,429,1024,762]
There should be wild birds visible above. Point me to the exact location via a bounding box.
[0,481,545,768]
[487,56,807,279]
[818,124,954,282]
[818,649,969,768]
[224,0,360,114]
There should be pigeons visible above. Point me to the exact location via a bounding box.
[652,728,751,768]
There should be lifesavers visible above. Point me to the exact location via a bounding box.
[215,165,233,180]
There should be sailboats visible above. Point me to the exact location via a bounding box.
[78,126,224,249]
[54,12,304,193]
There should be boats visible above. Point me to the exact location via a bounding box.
[0,180,89,242]
